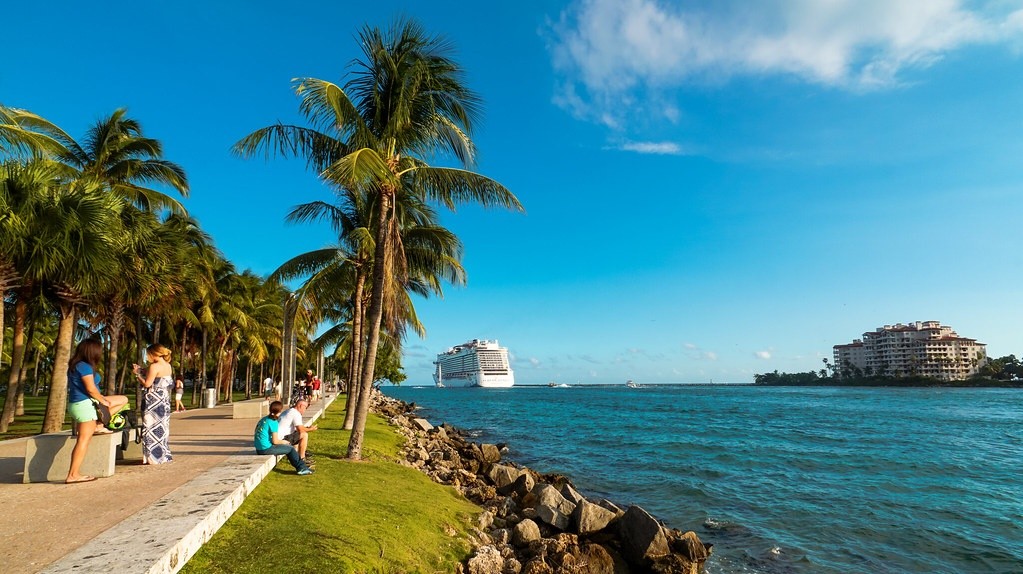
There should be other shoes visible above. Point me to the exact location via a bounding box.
[296,468,313,475]
[305,464,311,469]
[303,458,316,465]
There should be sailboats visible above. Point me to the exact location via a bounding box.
[437,363,445,388]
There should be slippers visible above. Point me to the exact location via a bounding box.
[93,428,113,435]
[65,476,98,484]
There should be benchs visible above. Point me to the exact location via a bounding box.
[22,428,144,484]
[233,399,269,419]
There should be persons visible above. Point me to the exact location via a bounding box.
[277,399,318,466]
[172,374,186,413]
[299,370,320,408]
[254,401,313,475]
[327,378,346,391]
[132,343,173,465]
[66,339,129,483]
[263,374,283,405]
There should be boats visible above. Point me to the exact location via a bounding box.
[547,382,556,387]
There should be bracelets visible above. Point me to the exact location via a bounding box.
[134,373,141,379]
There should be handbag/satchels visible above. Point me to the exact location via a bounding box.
[92,402,111,425]
[122,410,138,429]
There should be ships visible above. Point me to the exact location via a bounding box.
[432,339,514,387]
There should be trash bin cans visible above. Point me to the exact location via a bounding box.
[203,388,216,409]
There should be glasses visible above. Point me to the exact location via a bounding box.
[303,405,307,409]
